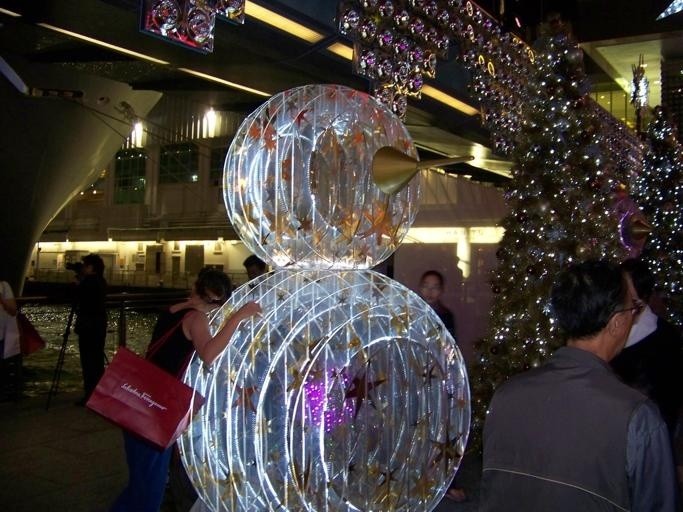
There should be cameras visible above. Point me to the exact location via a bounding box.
[65,261,84,273]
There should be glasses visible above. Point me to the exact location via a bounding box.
[606,300,644,325]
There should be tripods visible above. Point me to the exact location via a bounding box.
[45,306,110,411]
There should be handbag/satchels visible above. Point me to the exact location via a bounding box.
[16,312,45,355]
[86,310,205,450]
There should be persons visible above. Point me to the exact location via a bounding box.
[108,266,264,512]
[415,270,456,344]
[599,258,683,477]
[0,277,25,406]
[64,251,107,408]
[469,257,681,511]
[240,254,268,281]
